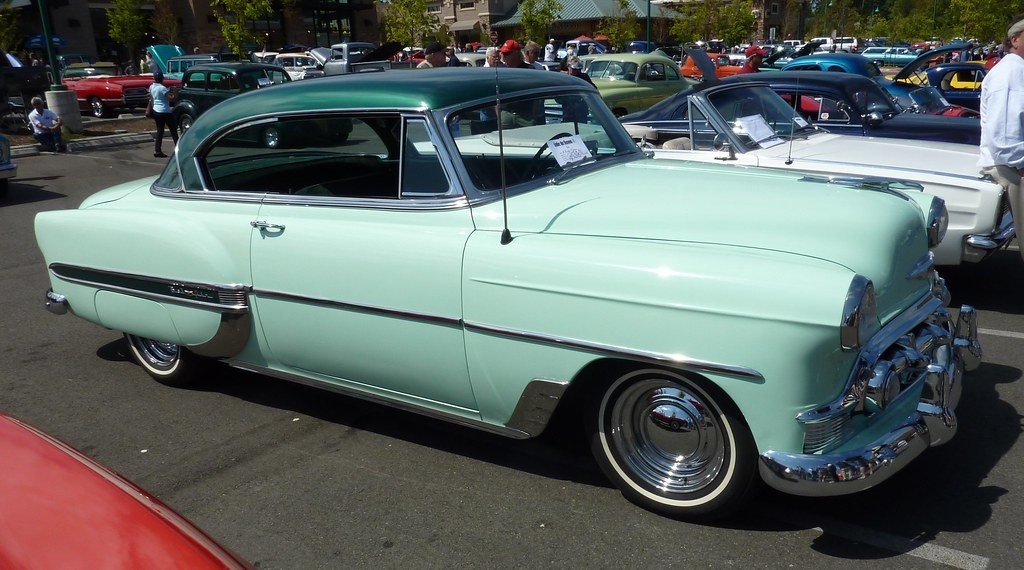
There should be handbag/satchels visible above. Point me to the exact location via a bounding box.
[145,86,153,118]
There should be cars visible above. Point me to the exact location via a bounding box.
[0,36,1009,148]
[0,412,257,570]
[0,135,17,201]
[51,67,183,118]
[34,68,984,522]
[542,52,699,124]
[378,78,1016,268]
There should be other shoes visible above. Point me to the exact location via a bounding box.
[154,152,168,158]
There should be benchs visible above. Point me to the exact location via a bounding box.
[223,155,415,197]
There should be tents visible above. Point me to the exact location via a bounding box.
[565,36,594,51]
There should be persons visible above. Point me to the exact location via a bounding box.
[416,41,460,140]
[499,39,537,131]
[980,20,1024,260]
[554,55,602,124]
[737,45,767,74]
[974,48,986,61]
[917,44,932,73]
[521,41,549,126]
[149,70,179,157]
[0,82,17,172]
[997,42,1004,59]
[587,46,596,56]
[960,47,970,61]
[448,49,461,67]
[125,60,140,77]
[29,52,42,97]
[28,97,64,152]
[560,50,574,75]
[194,47,201,55]
[146,51,158,74]
[544,38,555,62]
[718,44,748,66]
[941,52,952,63]
[39,57,51,68]
[140,55,150,74]
[829,44,837,53]
[985,47,997,60]
[480,46,503,133]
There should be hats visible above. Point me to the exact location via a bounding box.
[567,55,583,70]
[127,59,134,63]
[153,71,164,78]
[497,40,521,53]
[745,46,767,58]
[425,42,451,54]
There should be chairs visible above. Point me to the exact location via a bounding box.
[475,156,520,190]
[941,79,952,91]
[221,76,259,91]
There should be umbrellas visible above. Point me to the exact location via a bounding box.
[24,33,68,49]
[0,48,24,69]
